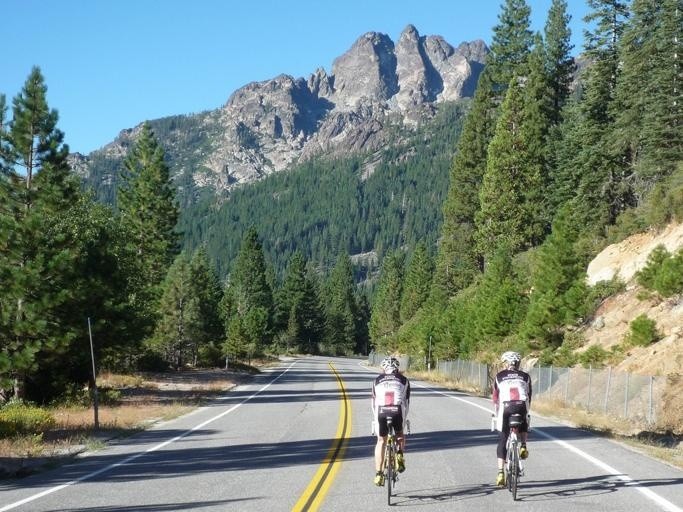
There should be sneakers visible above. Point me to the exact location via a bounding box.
[395,452,406,473]
[374,475,383,484]
[496,473,504,486]
[519,446,528,459]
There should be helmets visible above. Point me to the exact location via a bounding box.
[500,350,521,368]
[380,356,400,375]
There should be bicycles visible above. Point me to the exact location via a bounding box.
[490,415,530,500]
[370,418,410,506]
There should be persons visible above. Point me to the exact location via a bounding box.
[370,357,410,488]
[490,349,531,488]
